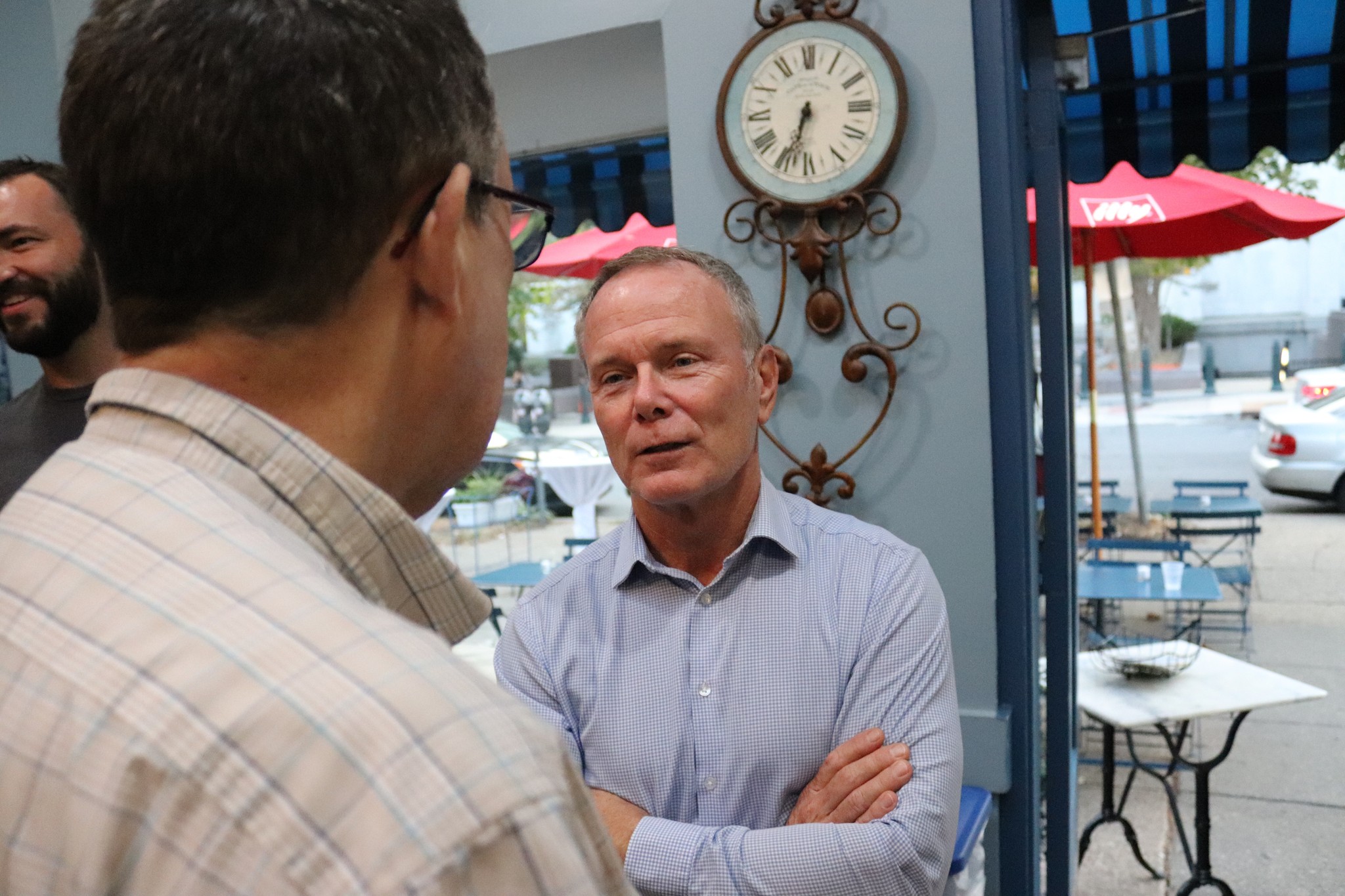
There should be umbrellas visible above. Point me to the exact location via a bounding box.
[1022,159,1345,635]
[517,214,676,279]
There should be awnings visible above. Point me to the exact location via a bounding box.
[1026,0,1345,187]
[510,131,676,239]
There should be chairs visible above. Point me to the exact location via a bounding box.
[1078,481,1253,652]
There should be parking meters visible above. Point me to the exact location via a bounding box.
[514,389,552,529]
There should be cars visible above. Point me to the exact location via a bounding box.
[443,422,611,515]
[1252,363,1345,508]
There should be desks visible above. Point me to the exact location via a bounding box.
[1036,496,1133,515]
[1076,563,1222,648]
[1040,640,1329,896]
[1169,496,1262,565]
[470,563,558,586]
[522,456,615,555]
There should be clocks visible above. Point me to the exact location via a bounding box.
[715,0,920,506]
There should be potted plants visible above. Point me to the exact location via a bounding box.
[1161,314,1197,365]
[452,467,520,526]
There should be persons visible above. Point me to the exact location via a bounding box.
[0,0,650,896]
[497,246,964,896]
[0,158,129,506]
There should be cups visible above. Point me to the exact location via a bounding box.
[1161,561,1185,590]
[540,560,552,575]
[1085,496,1092,506]
[1201,494,1211,507]
[1138,561,1151,582]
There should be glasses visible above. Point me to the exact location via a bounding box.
[388,176,557,274]
